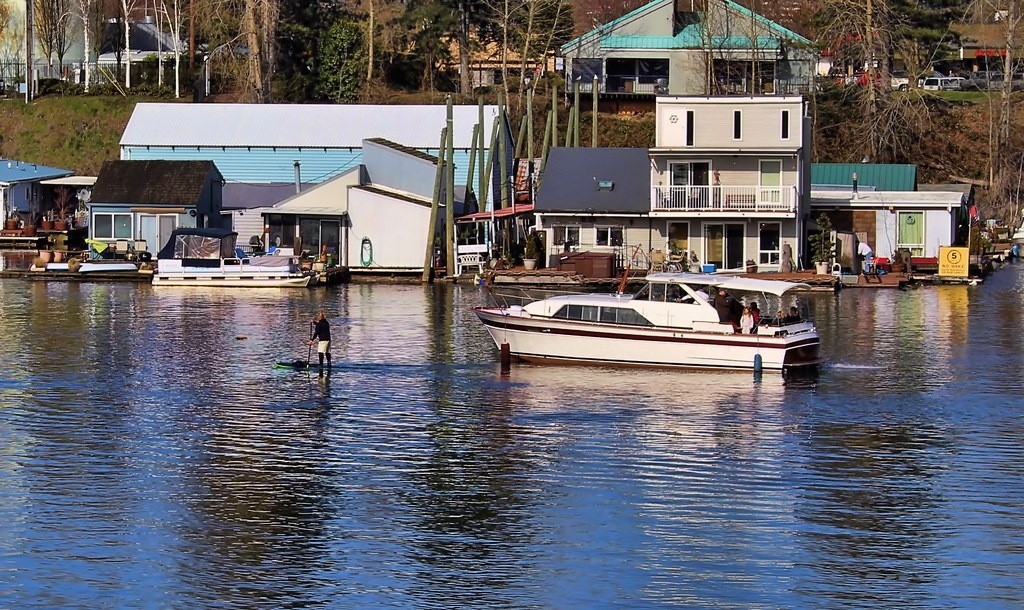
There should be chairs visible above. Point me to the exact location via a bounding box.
[266,246,280,257]
[113,239,146,263]
[863,264,881,284]
[650,249,686,273]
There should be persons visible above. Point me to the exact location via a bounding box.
[676,285,710,305]
[740,307,754,334]
[748,302,760,326]
[715,288,732,322]
[310,312,330,368]
[856,240,874,273]
[783,307,800,324]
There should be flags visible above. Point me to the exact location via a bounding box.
[474,275,485,285]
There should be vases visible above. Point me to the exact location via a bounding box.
[7,219,51,237]
[39,249,52,263]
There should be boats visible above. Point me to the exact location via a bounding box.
[152,227,321,287]
[471,244,831,369]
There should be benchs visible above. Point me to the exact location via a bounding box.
[726,194,756,208]
[58,251,91,262]
[757,319,813,337]
[692,321,737,335]
[457,254,485,274]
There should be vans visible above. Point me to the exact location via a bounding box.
[922,77,967,93]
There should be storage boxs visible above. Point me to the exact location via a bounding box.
[702,263,717,273]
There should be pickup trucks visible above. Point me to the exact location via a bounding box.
[889,73,924,92]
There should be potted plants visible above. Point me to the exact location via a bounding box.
[890,249,906,272]
[502,231,542,270]
[300,259,312,274]
[808,212,837,275]
[664,238,681,271]
[67,258,80,273]
[51,242,64,263]
[53,185,70,230]
[33,257,46,271]
